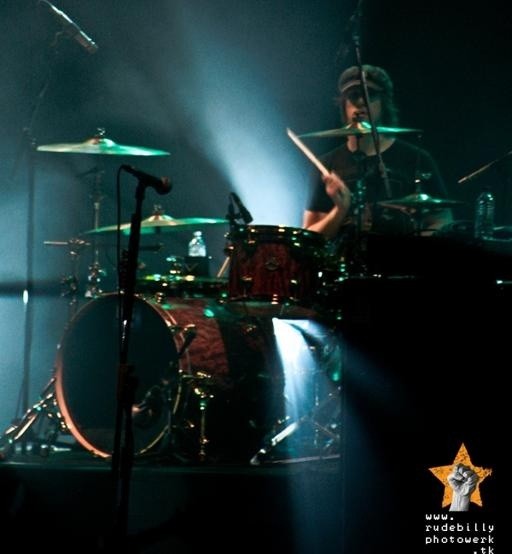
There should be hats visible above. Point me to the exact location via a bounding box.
[338,63,387,95]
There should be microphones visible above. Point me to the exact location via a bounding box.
[125,162,174,195]
[336,0,363,64]
[39,0,99,53]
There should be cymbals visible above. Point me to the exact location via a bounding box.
[300,121,425,140]
[38,137,170,157]
[379,194,465,215]
[82,212,228,236]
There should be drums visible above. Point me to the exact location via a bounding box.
[55,291,285,459]
[138,275,230,298]
[227,225,329,318]
[340,273,426,333]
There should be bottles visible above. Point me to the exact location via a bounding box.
[187,230,207,257]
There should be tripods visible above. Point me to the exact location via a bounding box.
[248,329,343,470]
[0,238,108,462]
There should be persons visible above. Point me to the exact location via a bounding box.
[446,463,479,514]
[301,62,455,241]
